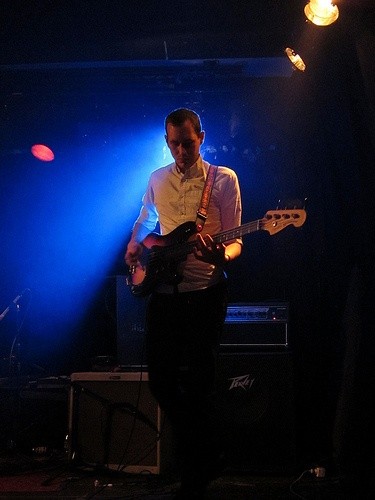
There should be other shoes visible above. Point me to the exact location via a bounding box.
[175,480,207,500]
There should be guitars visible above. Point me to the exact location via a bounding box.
[125,197,307,300]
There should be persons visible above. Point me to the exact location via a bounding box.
[126,107,243,487]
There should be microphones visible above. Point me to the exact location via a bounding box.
[0,294,22,321]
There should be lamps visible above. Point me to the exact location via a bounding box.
[283,31,322,73]
[302,0,346,29]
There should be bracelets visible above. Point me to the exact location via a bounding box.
[226,253,231,262]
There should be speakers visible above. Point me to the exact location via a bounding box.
[206,350,295,476]
[69,372,166,475]
